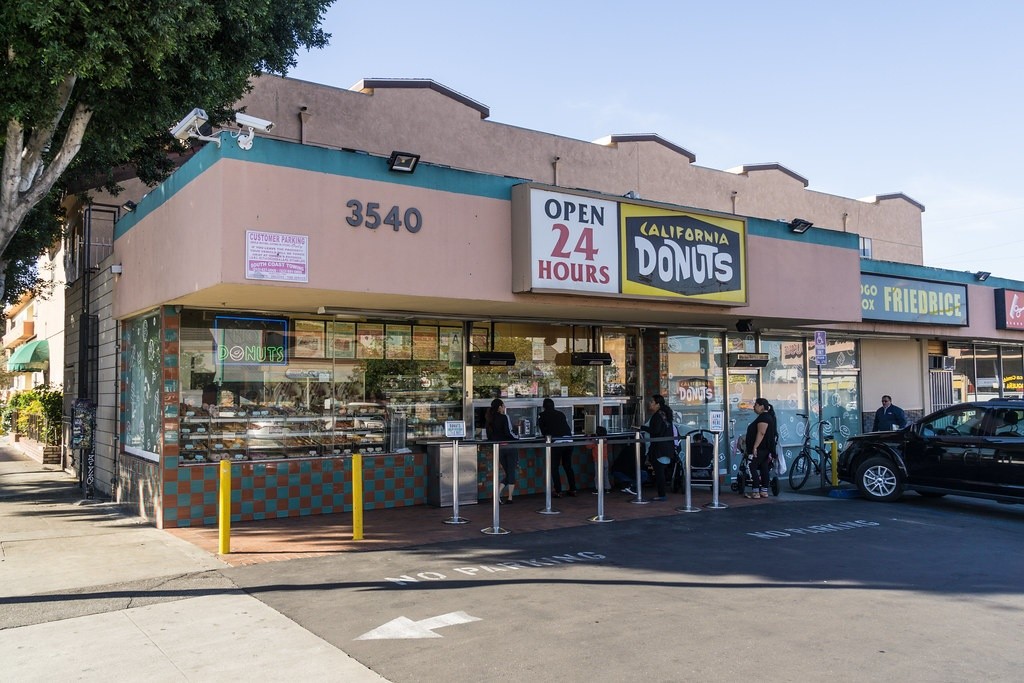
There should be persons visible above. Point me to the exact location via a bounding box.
[692,433,708,443]
[584,426,611,494]
[872,395,907,432]
[631,394,675,501]
[745,398,777,499]
[538,398,577,497]
[242,391,259,413]
[486,399,519,504]
[810,393,851,455]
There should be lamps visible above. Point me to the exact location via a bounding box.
[386,150,421,174]
[122,200,137,212]
[789,218,813,233]
[975,271,991,282]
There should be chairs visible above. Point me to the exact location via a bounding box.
[969,421,981,436]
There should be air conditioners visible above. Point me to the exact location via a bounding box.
[937,356,956,370]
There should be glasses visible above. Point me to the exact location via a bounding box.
[882,401,890,402]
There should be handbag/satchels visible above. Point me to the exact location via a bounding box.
[771,439,787,477]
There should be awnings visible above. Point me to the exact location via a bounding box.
[7,340,49,373]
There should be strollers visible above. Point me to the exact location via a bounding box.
[730,432,782,496]
[677,429,719,492]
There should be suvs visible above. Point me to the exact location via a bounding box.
[837,400,1024,504]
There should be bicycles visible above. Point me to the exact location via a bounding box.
[789,413,843,490]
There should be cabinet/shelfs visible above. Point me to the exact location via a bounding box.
[179,412,388,463]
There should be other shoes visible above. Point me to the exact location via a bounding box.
[653,496,667,501]
[621,486,637,495]
[744,492,760,498]
[592,489,598,494]
[499,497,505,505]
[507,498,513,504]
[605,489,610,493]
[760,491,768,498]
[567,489,577,496]
[552,491,563,498]
